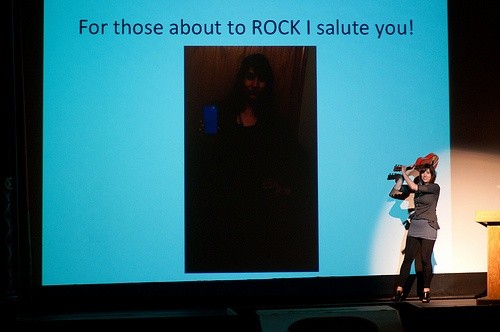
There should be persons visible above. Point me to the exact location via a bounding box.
[385,160,440,305]
[185,53,318,272]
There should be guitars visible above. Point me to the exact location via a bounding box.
[394,153,439,172]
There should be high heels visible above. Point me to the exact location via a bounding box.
[392,291,404,301]
[421,291,431,303]
